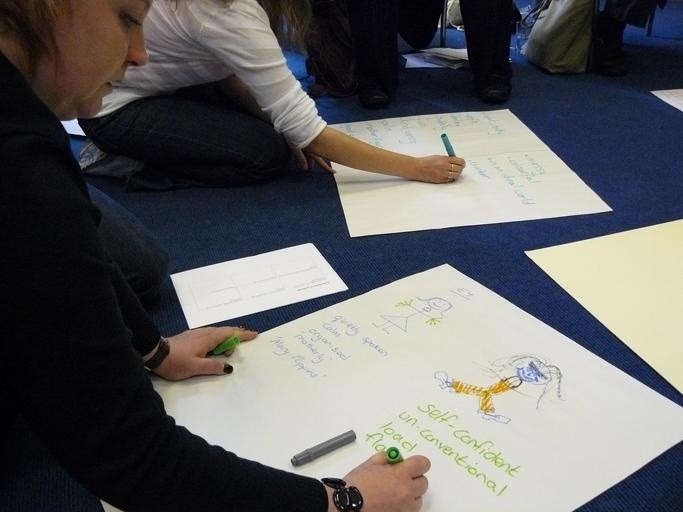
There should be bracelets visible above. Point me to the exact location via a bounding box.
[322,475,363,511]
[143,334,170,370]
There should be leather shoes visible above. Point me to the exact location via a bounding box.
[474,69,513,103]
[355,70,389,109]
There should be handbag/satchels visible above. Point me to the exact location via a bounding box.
[520,0,595,75]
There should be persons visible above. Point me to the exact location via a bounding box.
[76,0,467,191]
[1,0,431,511]
[349,1,522,108]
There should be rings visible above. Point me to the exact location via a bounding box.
[448,172,455,179]
[449,157,455,163]
[451,165,454,170]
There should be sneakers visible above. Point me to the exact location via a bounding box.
[77,144,144,180]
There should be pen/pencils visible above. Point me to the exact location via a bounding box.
[441,134,456,157]
[291,430,357,466]
[386,447,403,464]
